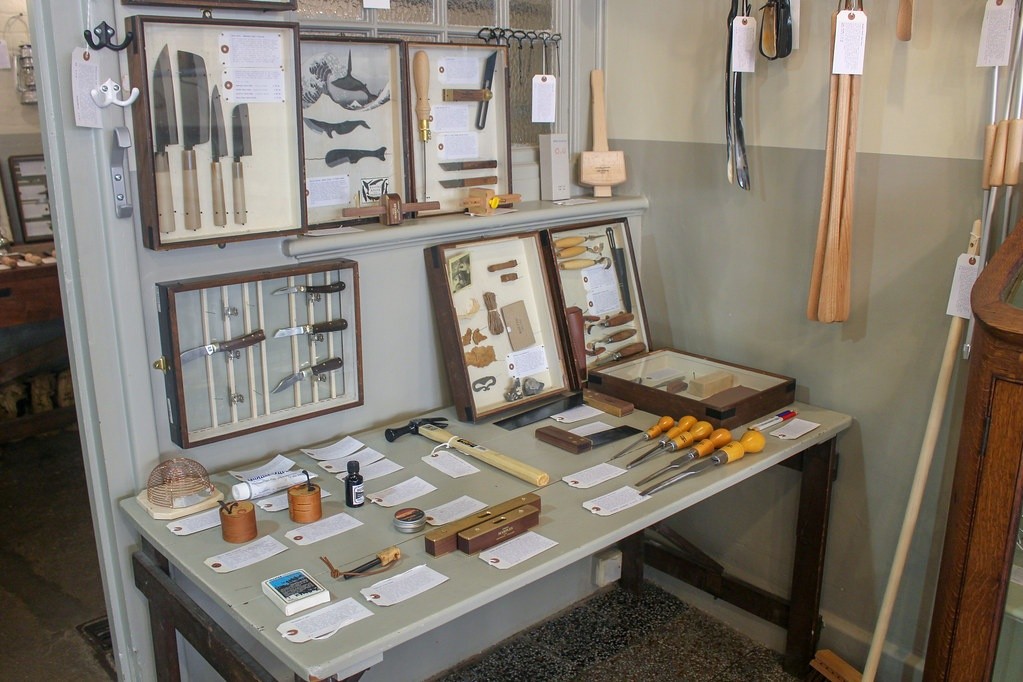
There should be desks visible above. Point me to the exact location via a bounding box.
[117,401,852,682]
[0,240,77,446]
[922,214,1023,682]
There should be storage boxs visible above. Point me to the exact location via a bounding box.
[539,133,571,202]
[154,258,364,449]
[9,154,54,244]
[539,216,797,431]
[405,41,513,217]
[126,14,306,250]
[419,229,571,423]
[300,34,413,230]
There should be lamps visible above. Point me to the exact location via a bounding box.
[15,45,38,104]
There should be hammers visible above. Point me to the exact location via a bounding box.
[384,414,552,488]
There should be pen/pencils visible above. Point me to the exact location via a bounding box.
[748,409,799,431]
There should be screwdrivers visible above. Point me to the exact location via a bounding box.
[594,342,646,366]
[556,233,607,249]
[414,50,431,202]
[606,414,767,497]
